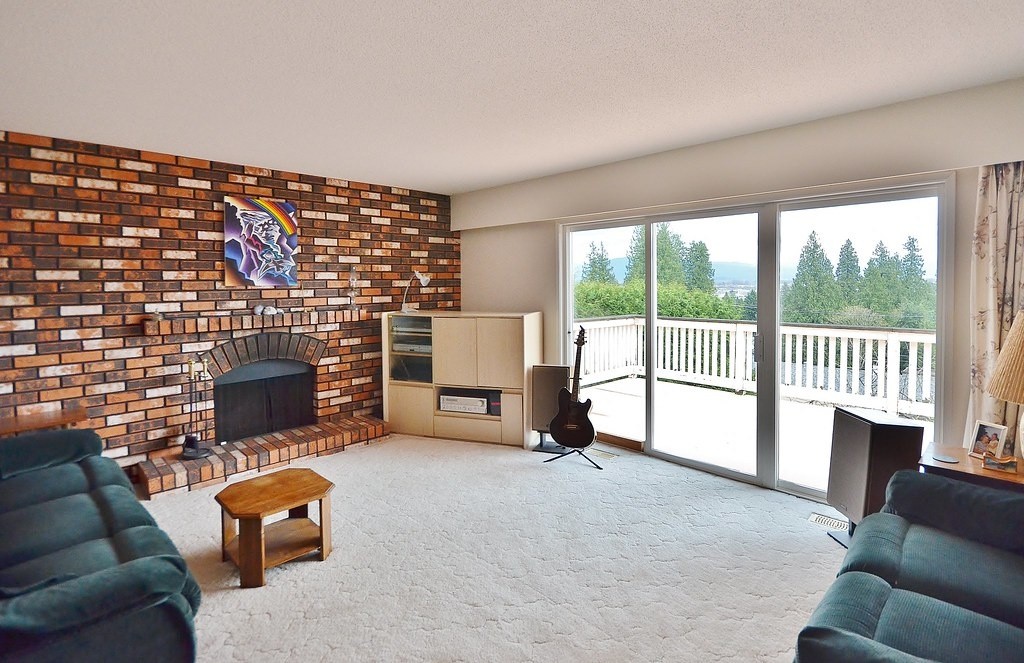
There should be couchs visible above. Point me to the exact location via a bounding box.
[0,428,202,663]
[794,470,1024,663]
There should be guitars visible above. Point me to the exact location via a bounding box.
[549,324,597,448]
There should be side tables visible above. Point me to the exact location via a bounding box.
[918,441,1024,492]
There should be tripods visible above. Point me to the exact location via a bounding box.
[542,450,604,469]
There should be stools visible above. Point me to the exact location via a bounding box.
[213,468,336,588]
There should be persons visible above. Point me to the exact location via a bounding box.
[974,432,999,456]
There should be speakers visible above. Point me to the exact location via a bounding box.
[532,364,575,455]
[825,407,925,549]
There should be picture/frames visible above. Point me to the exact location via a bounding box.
[968,420,1009,460]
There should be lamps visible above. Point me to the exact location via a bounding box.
[401,270,430,312]
[985,309,1024,455]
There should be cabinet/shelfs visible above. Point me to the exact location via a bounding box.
[531,364,571,456]
[381,310,544,450]
[826,404,925,549]
